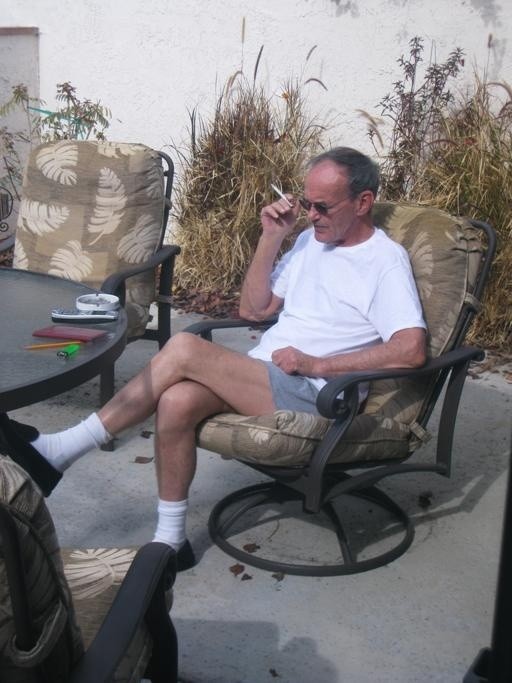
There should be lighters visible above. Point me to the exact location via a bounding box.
[57,344,80,357]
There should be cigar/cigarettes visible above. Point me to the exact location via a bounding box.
[96,293,99,302]
[271,184,293,208]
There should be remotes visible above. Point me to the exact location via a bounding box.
[52,309,119,323]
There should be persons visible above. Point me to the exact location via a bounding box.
[0,147,427,573]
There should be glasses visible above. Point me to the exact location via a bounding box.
[299,193,357,214]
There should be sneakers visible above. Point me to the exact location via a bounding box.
[0,411,62,497]
[176,539,194,570]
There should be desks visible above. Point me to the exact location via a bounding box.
[0,268,128,413]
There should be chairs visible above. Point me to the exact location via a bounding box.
[0,453,177,682]
[180,202,494,575]
[9,140,181,451]
